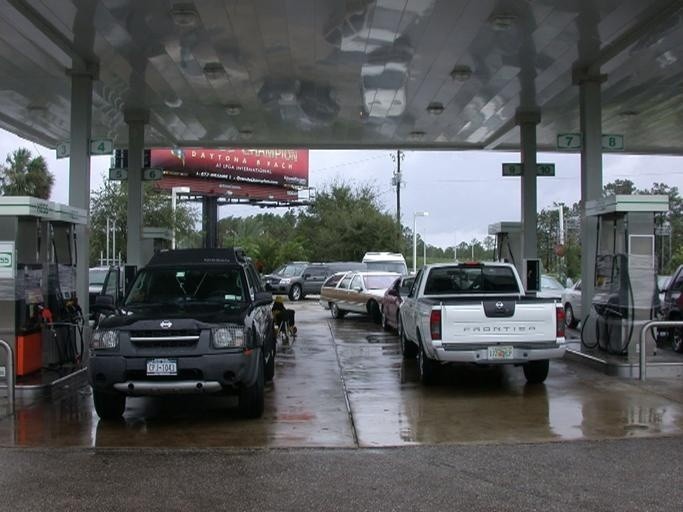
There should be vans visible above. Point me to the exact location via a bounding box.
[285,262,361,301]
[361,252,408,276]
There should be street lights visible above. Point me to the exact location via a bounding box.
[411,209,431,273]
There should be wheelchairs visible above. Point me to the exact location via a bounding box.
[271,307,295,347]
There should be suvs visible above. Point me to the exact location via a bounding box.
[88,249,276,419]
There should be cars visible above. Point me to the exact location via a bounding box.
[262,262,309,295]
[381,274,417,334]
[320,271,399,325]
[536,275,566,296]
[562,277,583,330]
[256,1,436,126]
[88,265,123,304]
[656,264,683,352]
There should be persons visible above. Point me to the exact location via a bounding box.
[256,259,265,273]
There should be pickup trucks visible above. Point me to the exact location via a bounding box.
[396,260,567,384]
